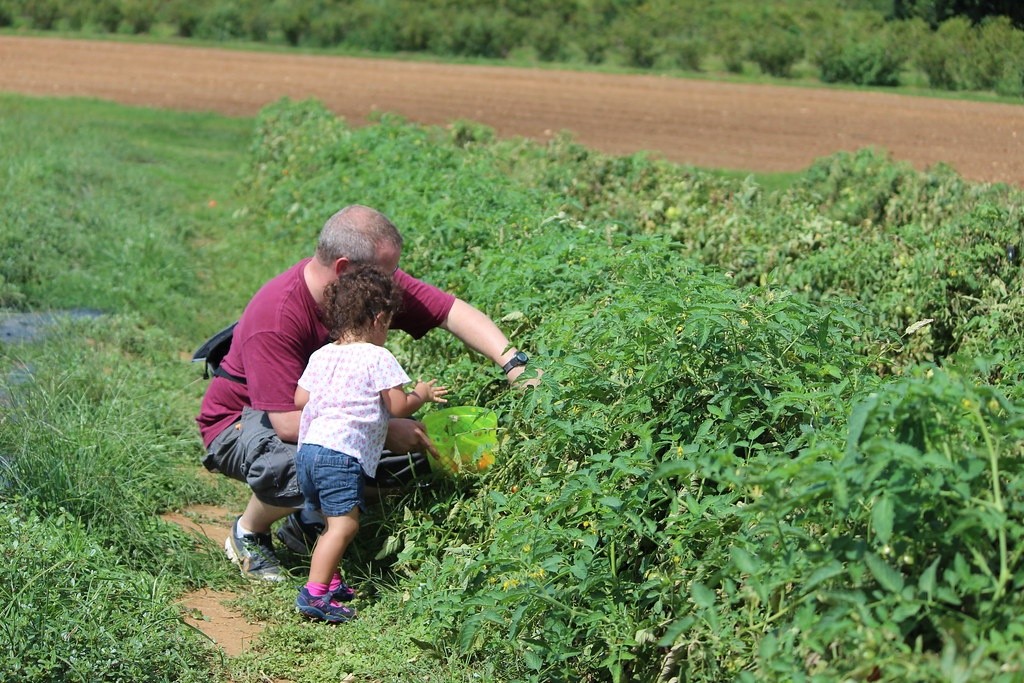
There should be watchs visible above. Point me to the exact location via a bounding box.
[502,351,530,375]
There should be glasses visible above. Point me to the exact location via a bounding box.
[391,264,399,275]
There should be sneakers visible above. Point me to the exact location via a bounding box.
[330,582,358,602]
[294,585,356,623]
[275,509,324,555]
[225,514,288,584]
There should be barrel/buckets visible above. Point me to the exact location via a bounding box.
[421,406,497,475]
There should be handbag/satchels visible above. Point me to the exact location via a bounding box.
[191,321,239,380]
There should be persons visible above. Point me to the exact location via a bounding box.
[196,204,565,624]
